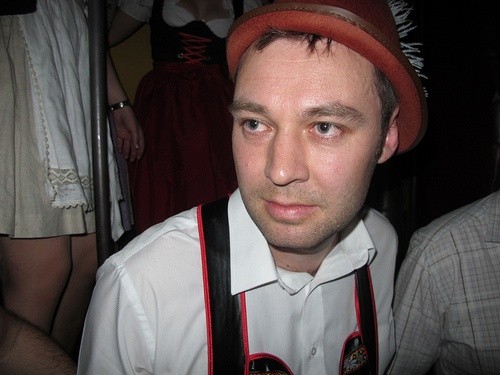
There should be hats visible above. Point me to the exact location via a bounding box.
[226,0,428,154]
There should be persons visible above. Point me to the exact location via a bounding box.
[0,0,146,375]
[76,0,427,375]
[106,0,272,239]
[382,190,500,375]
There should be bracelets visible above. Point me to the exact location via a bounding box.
[109,99,131,114]
